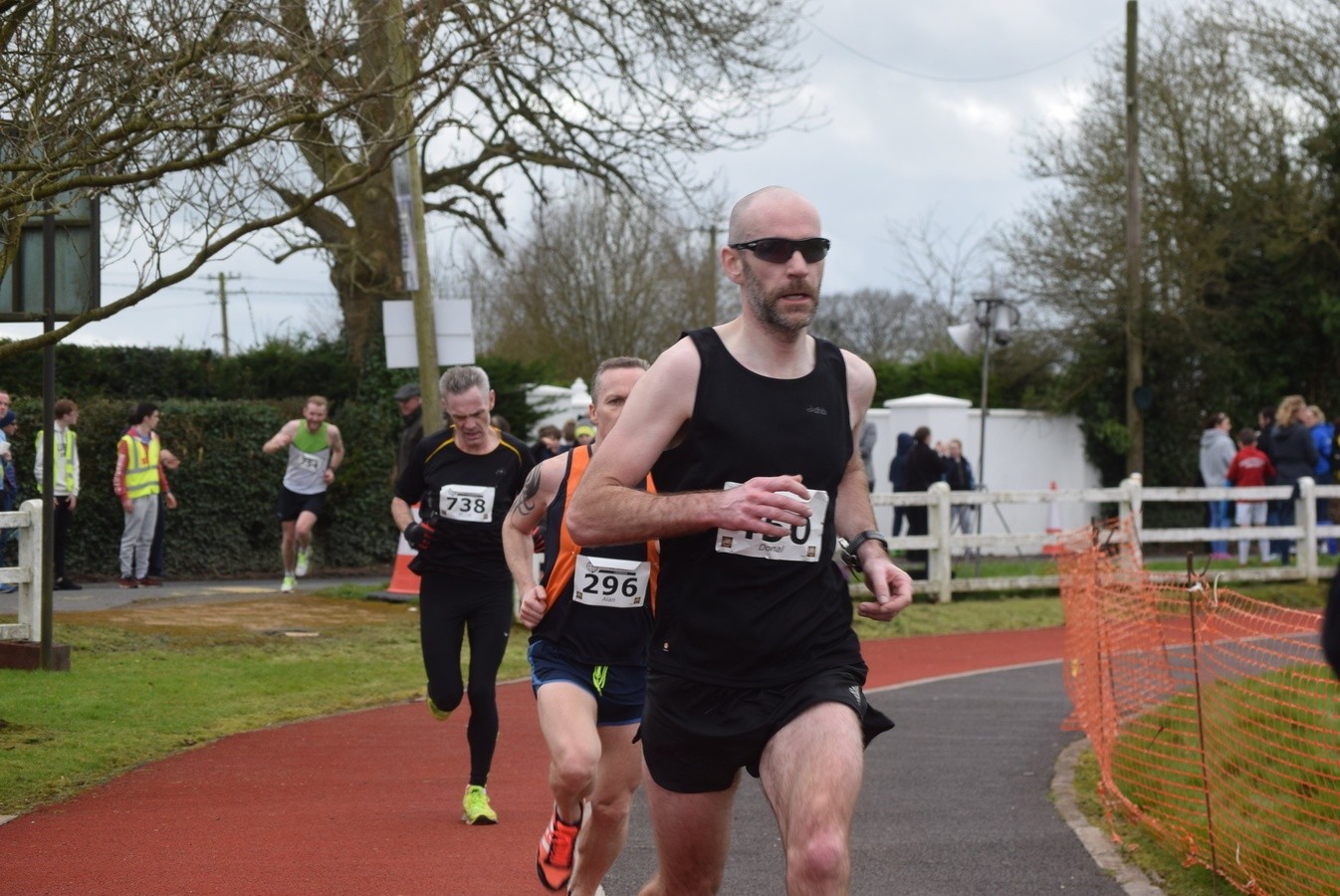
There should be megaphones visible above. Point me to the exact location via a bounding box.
[946,315,986,356]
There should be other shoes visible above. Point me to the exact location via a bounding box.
[1211,552,1232,559]
[0,584,18,594]
[52,578,82,591]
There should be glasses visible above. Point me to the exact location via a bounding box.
[730,237,831,265]
[9,422,16,426]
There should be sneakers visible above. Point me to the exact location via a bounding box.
[460,782,496,825]
[295,543,311,577]
[279,576,295,592]
[536,795,591,889]
[428,697,451,720]
[119,577,137,589]
[136,576,162,589]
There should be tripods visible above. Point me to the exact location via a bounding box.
[952,299,1026,577]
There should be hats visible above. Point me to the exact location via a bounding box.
[573,419,594,441]
[393,385,420,401]
[0,411,16,428]
[1234,428,1261,442]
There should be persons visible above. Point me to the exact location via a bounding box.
[114,402,180,588]
[859,418,975,562]
[1198,396,1336,566]
[0,390,18,593]
[502,356,660,896]
[563,184,914,896]
[392,383,600,566]
[263,396,344,593]
[34,399,81,590]
[391,368,535,826]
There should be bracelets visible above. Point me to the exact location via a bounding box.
[840,530,890,573]
[327,466,337,474]
